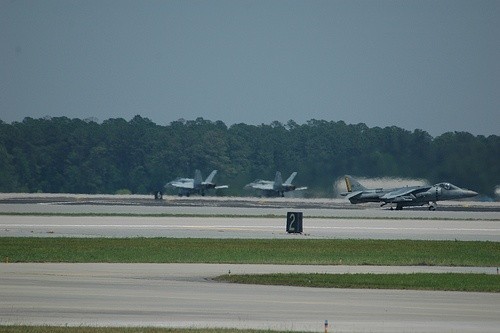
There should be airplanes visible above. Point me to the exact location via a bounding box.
[340,175,479,211]
[244,170,308,197]
[164,169,229,197]
